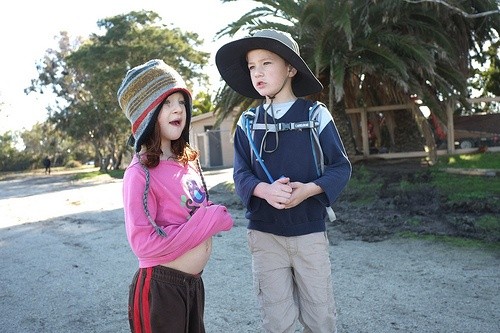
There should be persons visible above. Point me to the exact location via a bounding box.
[117,58,233,333]
[44,155,51,174]
[233,28,353,333]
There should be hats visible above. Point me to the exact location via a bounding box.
[215,28,324,100]
[117,58,192,153]
